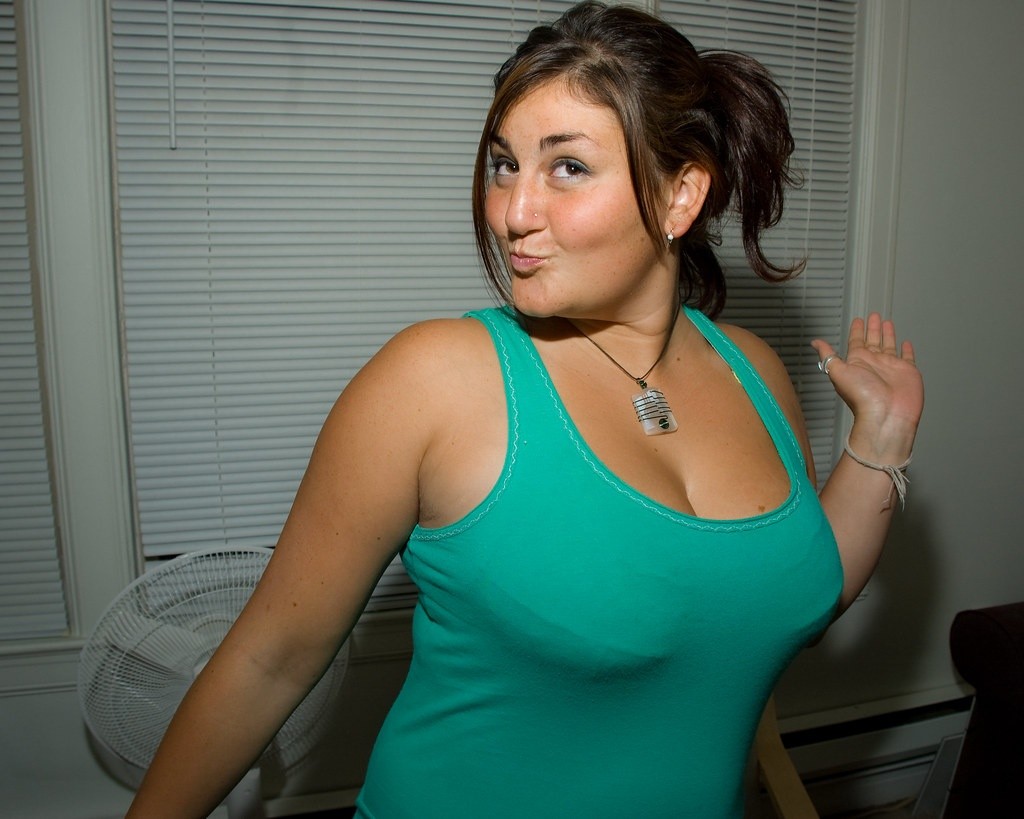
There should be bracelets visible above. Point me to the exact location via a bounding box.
[844,438,912,511]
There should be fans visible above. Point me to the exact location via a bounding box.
[77,544,351,819]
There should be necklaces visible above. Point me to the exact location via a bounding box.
[568,300,682,435]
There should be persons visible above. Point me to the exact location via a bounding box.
[122,0,925,819]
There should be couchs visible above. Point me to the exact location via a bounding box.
[943,604,1024,819]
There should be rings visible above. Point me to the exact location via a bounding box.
[818,354,838,374]
